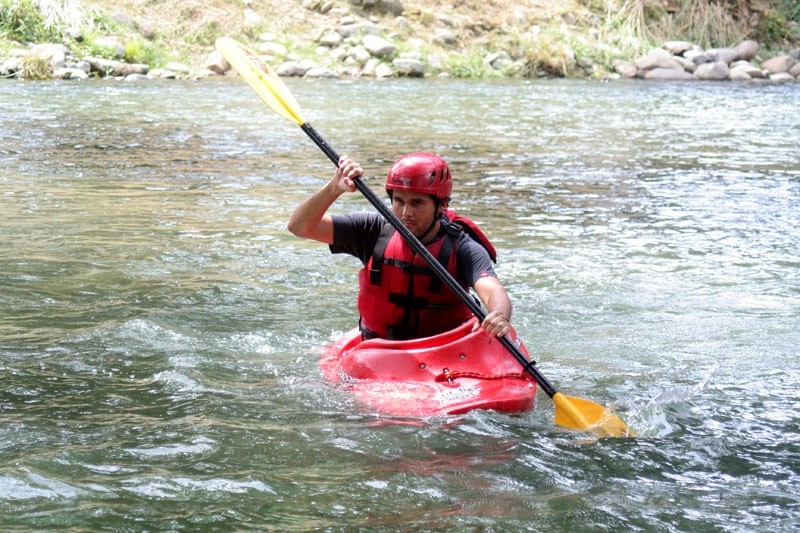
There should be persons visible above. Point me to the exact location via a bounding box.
[291,155,511,338]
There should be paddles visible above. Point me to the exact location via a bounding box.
[214,37,609,427]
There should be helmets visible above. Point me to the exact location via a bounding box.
[385,152,453,200]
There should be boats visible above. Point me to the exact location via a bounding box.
[319,314,538,425]
